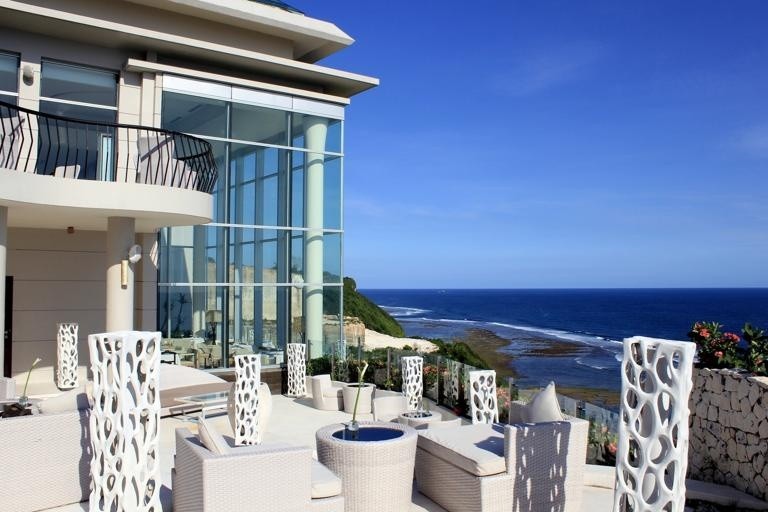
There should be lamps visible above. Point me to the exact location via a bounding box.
[206,310,222,345]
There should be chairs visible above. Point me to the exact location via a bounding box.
[0,411,93,512]
[164,339,270,368]
[416,401,590,511]
[172,427,344,512]
[372,388,409,421]
[312,372,345,412]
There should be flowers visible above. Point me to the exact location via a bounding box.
[20,357,42,400]
[350,360,368,423]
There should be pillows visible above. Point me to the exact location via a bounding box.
[36,385,89,416]
[198,417,231,453]
[520,382,562,424]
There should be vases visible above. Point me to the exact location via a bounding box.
[348,420,359,431]
[18,396,29,407]
